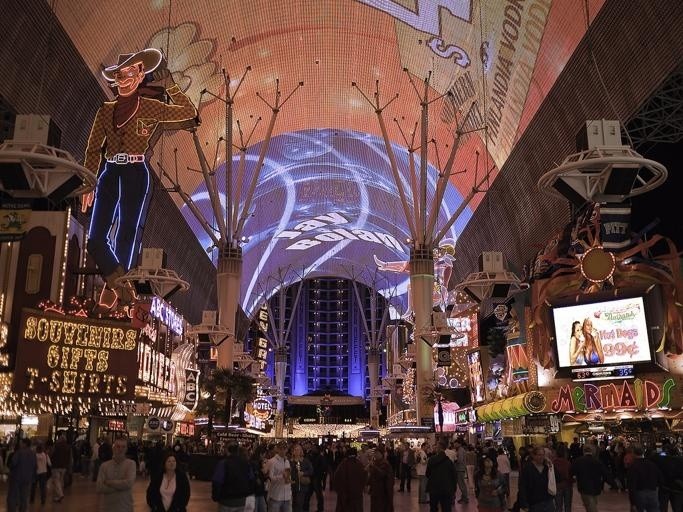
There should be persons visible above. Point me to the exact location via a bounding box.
[80,47,200,323]
[568,320,602,366]
